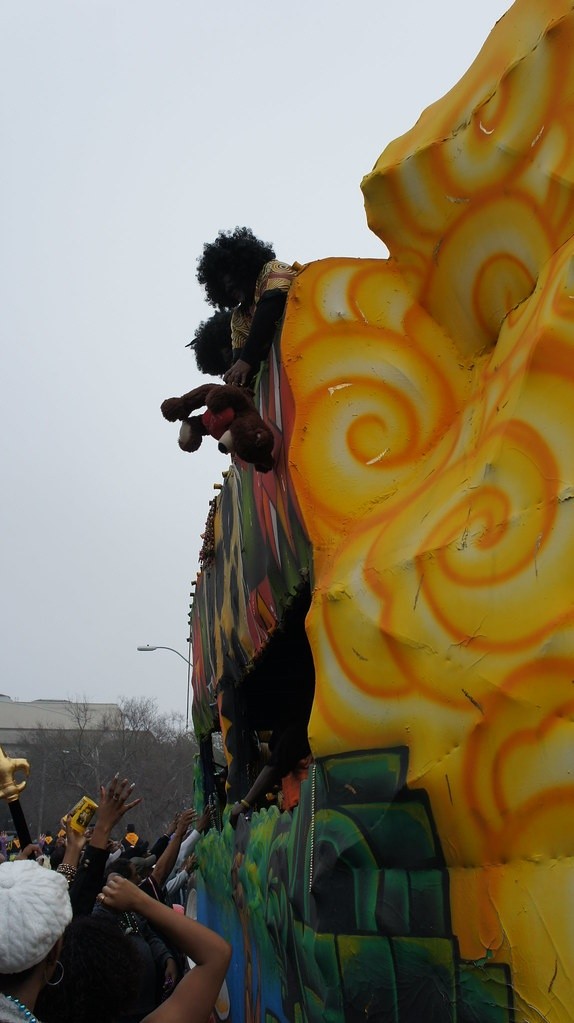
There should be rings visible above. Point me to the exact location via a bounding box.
[98,894,105,903]
[173,820,175,823]
[113,796,118,800]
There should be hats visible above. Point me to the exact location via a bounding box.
[127,824,135,832]
[130,854,156,873]
[46,831,51,835]
[0,860,73,973]
[105,848,121,868]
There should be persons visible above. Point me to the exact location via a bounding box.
[225,719,315,829]
[0,772,233,1023]
[185,227,297,387]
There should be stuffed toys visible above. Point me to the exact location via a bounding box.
[161,383,274,473]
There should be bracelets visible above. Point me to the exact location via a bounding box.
[57,863,77,877]
[241,800,250,808]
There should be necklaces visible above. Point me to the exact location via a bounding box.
[5,994,37,1023]
[123,911,138,934]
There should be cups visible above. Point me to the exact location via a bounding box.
[62,796,99,834]
